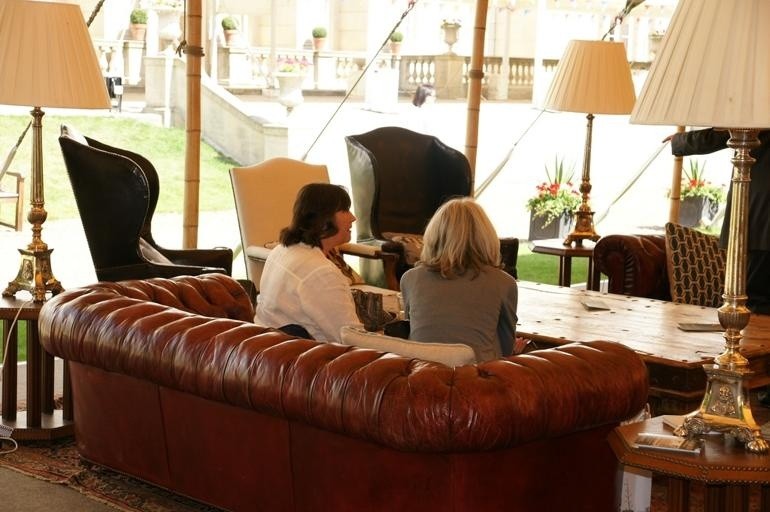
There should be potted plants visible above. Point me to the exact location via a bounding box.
[130,9,147,38]
[313,28,325,48]
[391,30,400,54]
[222,17,238,45]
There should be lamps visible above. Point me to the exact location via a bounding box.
[0,0,114,304]
[629,0,768,452]
[533,39,637,245]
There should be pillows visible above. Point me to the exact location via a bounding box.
[664,222,728,308]
[383,228,425,264]
[265,240,363,287]
[342,325,474,372]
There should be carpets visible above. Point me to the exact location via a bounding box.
[2,390,215,512]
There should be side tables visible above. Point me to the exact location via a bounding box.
[608,408,770,511]
[0,294,75,443]
[529,238,596,287]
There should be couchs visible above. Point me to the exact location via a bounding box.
[37,274,651,511]
[590,234,670,302]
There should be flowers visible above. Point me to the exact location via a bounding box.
[525,155,583,232]
[668,158,728,208]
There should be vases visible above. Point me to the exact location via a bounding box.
[529,204,563,243]
[675,197,705,232]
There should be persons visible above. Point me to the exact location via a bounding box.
[400,197,531,363]
[659,125,770,318]
[253,182,387,337]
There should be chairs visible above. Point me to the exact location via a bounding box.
[346,126,519,291]
[58,123,233,286]
[228,157,402,318]
